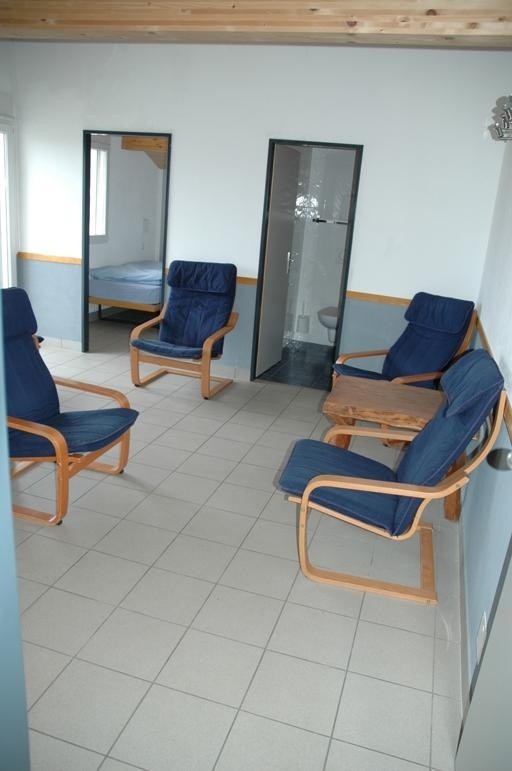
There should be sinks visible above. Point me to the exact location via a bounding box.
[317,306,339,329]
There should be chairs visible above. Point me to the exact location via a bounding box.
[1,284,139,528]
[129,258,241,401]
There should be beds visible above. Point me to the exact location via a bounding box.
[89,260,163,328]
